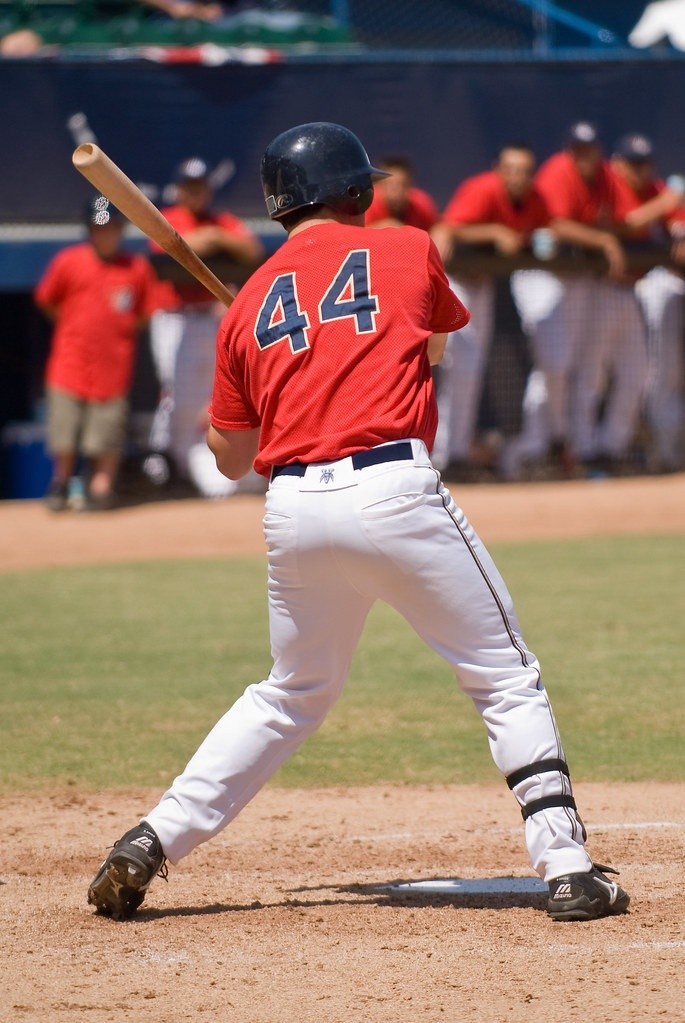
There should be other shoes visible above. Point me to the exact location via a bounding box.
[451,451,675,484]
[49,465,121,511]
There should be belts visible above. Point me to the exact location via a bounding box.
[272,441,414,479]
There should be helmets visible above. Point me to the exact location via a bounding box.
[263,122,391,216]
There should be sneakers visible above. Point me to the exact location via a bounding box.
[88,822,165,921]
[547,863,630,920]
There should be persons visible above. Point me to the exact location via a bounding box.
[0,0,223,59]
[36,124,685,512]
[86,122,630,921]
[628,0,685,51]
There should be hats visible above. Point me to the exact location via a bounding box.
[567,120,600,149]
[617,131,654,162]
[174,155,209,184]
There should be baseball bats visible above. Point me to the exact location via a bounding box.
[71,140,235,310]
